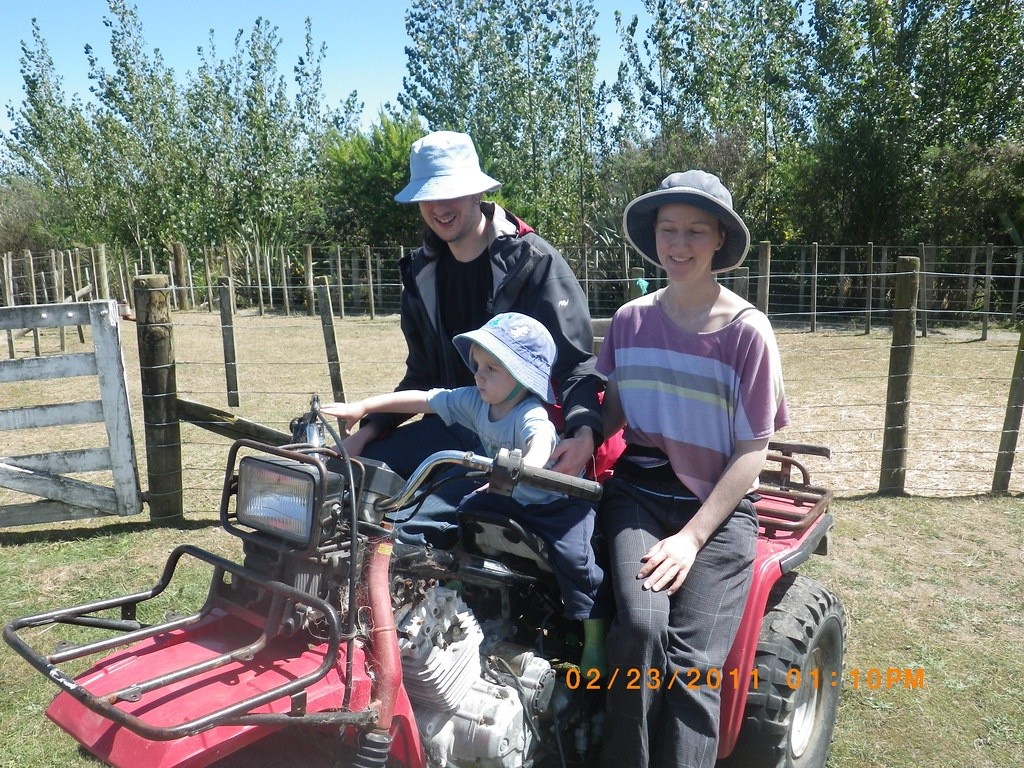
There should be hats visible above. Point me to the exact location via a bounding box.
[394,130,502,203]
[621,169,751,274]
[451,312,559,405]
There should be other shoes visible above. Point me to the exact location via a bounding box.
[563,621,583,649]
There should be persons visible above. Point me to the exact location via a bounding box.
[558,170,788,768]
[320,131,603,649]
